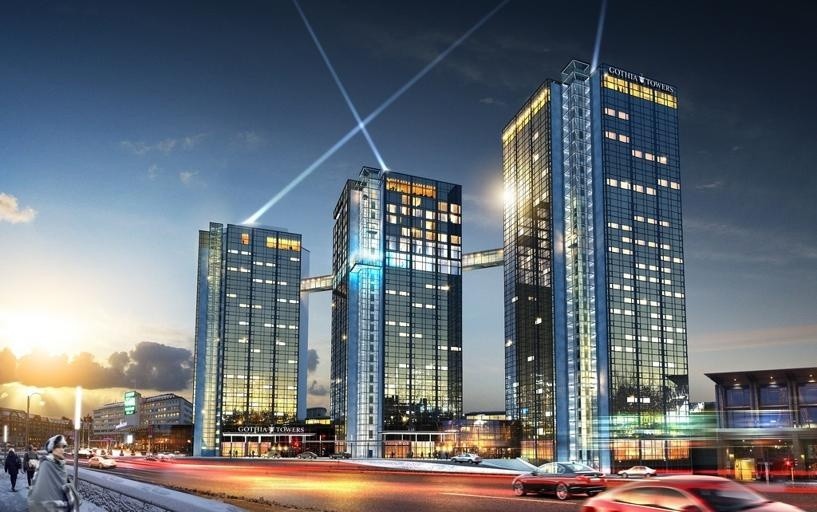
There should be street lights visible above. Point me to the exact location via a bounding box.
[3,425,7,458]
[26,392,45,445]
[73,386,82,512]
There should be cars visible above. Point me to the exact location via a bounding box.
[512,462,807,512]
[297,451,317,460]
[451,453,482,464]
[88,456,117,469]
[261,451,281,459]
[329,452,352,459]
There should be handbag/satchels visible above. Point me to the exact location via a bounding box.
[28,459,39,468]
